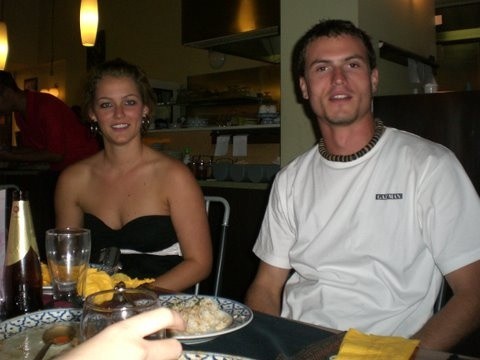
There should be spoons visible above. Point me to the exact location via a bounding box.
[35,325,75,360]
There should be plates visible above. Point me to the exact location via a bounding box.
[82,295,254,344]
[0,308,116,360]
[179,351,257,360]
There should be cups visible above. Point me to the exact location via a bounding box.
[46,227,91,302]
[81,288,168,340]
[197,155,212,180]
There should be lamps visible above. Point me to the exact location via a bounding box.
[80,0,98,47]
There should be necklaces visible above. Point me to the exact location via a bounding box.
[318,117,384,162]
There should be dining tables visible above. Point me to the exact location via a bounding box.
[1,288,474,359]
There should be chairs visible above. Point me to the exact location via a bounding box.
[148,195,230,297]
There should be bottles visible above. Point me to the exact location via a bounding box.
[182,147,193,173]
[5,190,43,317]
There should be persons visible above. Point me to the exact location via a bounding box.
[55,59,212,294]
[0,71,99,172]
[244,18,480,352]
